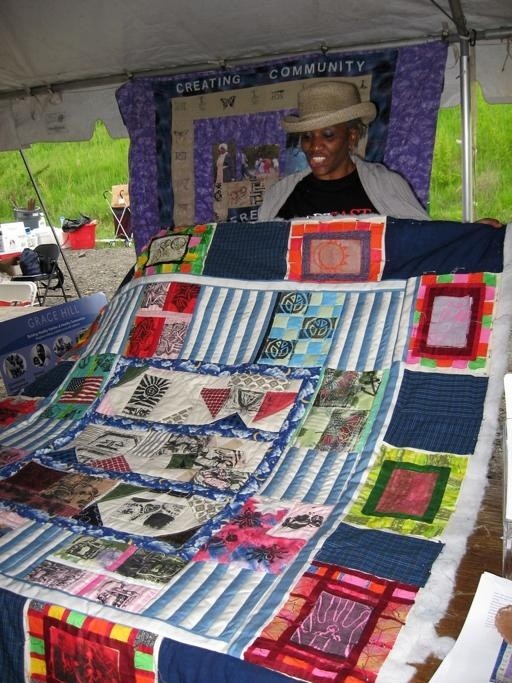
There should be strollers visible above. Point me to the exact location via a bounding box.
[103,190,131,248]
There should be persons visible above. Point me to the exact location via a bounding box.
[257,81,503,232]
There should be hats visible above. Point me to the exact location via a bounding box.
[281,81,377,133]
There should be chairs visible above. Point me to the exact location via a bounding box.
[9,243,73,307]
[1,281,37,307]
